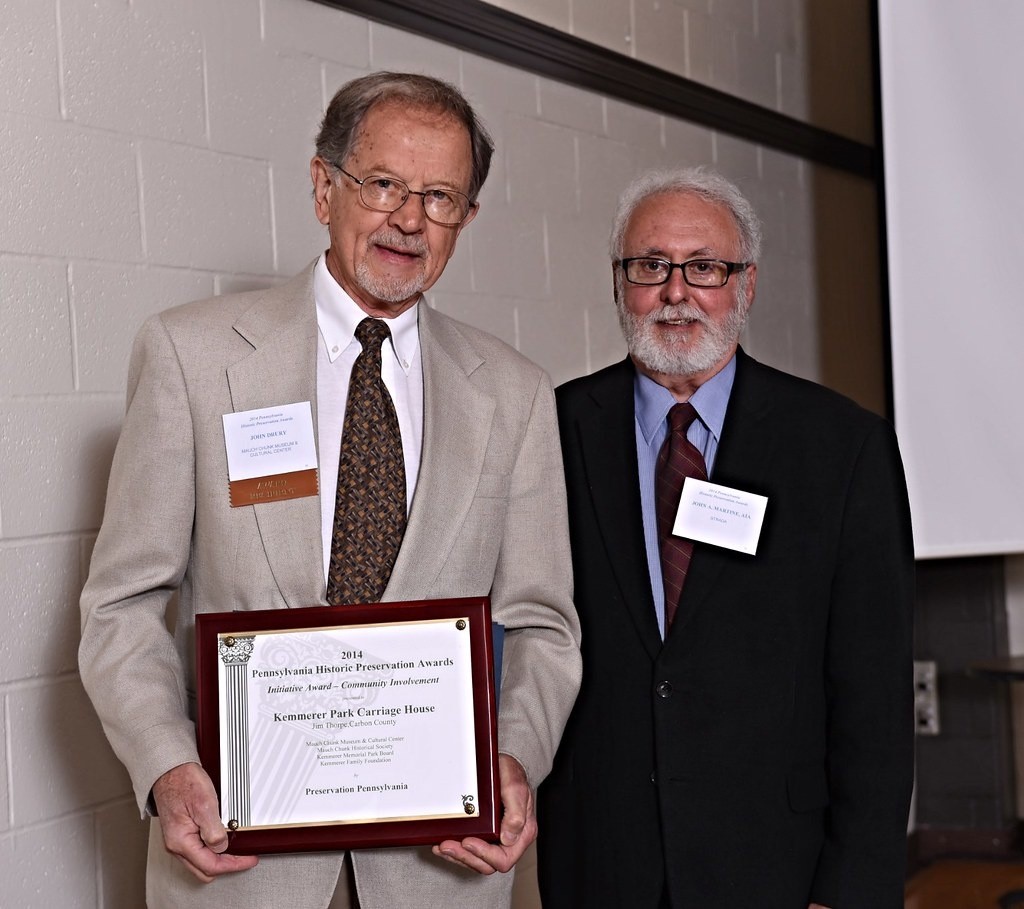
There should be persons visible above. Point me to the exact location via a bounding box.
[78,73,583,909]
[553,166,917,909]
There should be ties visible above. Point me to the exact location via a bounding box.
[655,404,708,641]
[327,318,407,604]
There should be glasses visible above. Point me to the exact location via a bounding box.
[617,256,746,287]
[334,163,476,227]
